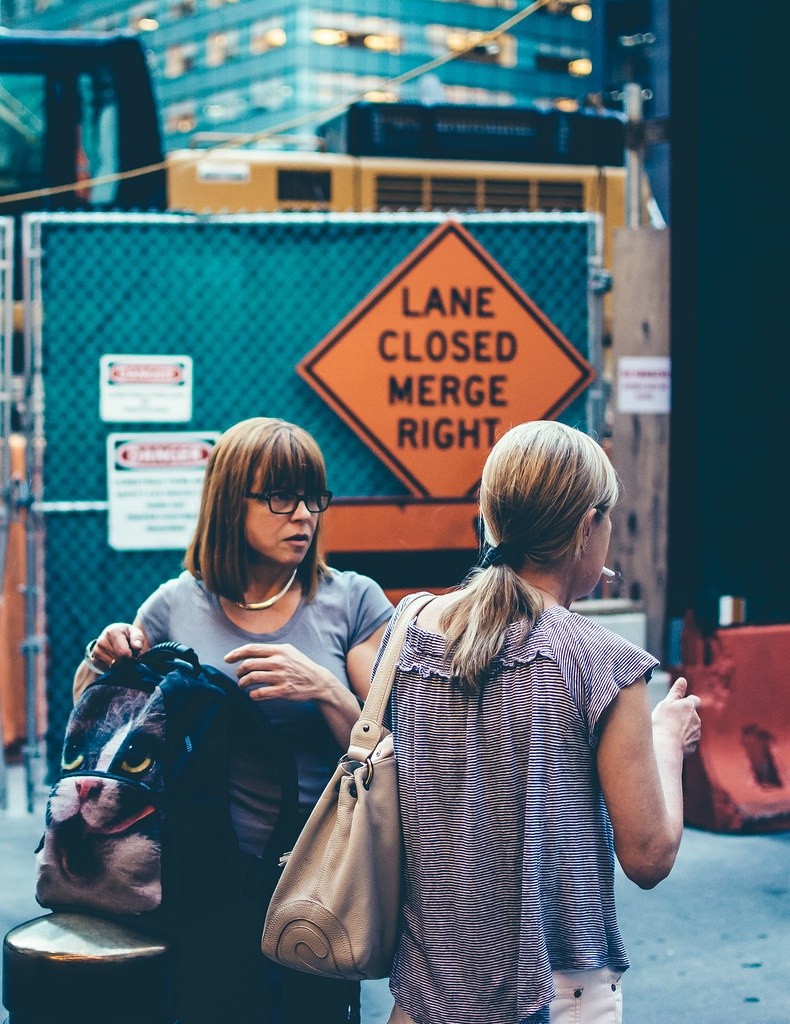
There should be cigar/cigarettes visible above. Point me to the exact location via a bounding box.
[600,563,616,580]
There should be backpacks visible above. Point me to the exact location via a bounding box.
[34,641,301,946]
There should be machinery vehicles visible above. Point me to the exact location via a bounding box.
[0,29,669,749]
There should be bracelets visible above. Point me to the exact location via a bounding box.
[83,636,116,676]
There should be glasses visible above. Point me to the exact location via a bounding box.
[244,490,333,515]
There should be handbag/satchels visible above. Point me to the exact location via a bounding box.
[259,591,433,981]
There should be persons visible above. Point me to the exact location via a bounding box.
[369,420,702,1024]
[72,415,398,1023]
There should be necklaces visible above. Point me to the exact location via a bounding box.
[225,563,299,610]
